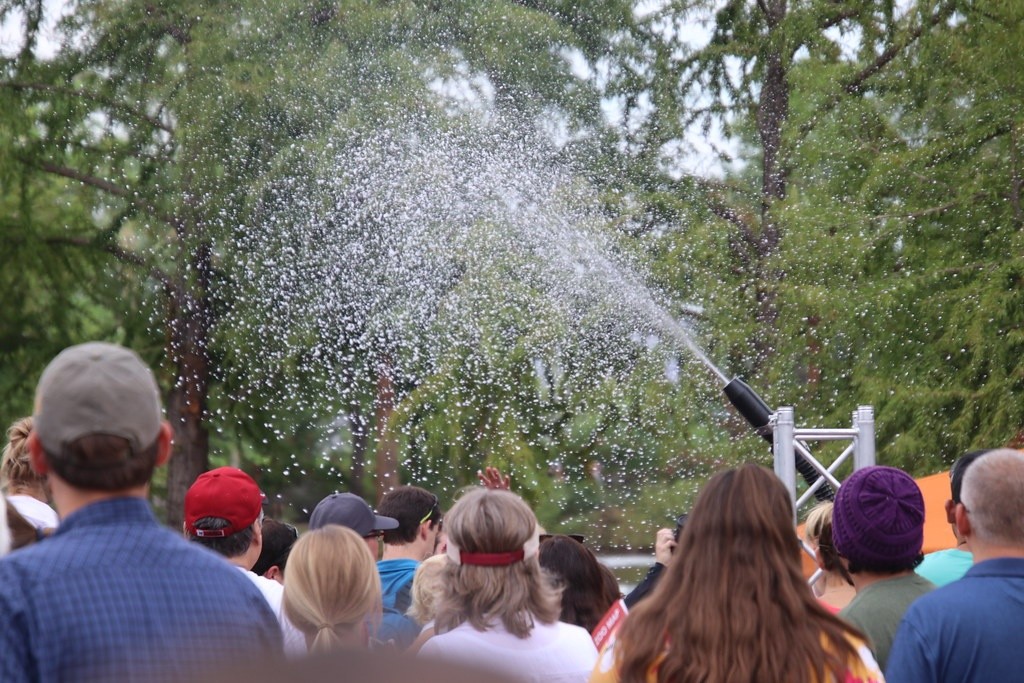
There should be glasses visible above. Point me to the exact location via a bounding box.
[421,494,439,524]
[538,534,584,549]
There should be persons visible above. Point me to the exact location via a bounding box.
[832,466,939,683]
[587,463,886,683]
[805,499,857,615]
[0,340,284,683]
[885,450,1024,683]
[914,449,989,590]
[0,418,684,683]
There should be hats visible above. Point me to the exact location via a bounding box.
[309,492,399,537]
[184,465,268,538]
[832,466,925,566]
[952,450,988,504]
[33,341,162,469]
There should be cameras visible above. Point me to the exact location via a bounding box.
[668,513,688,553]
